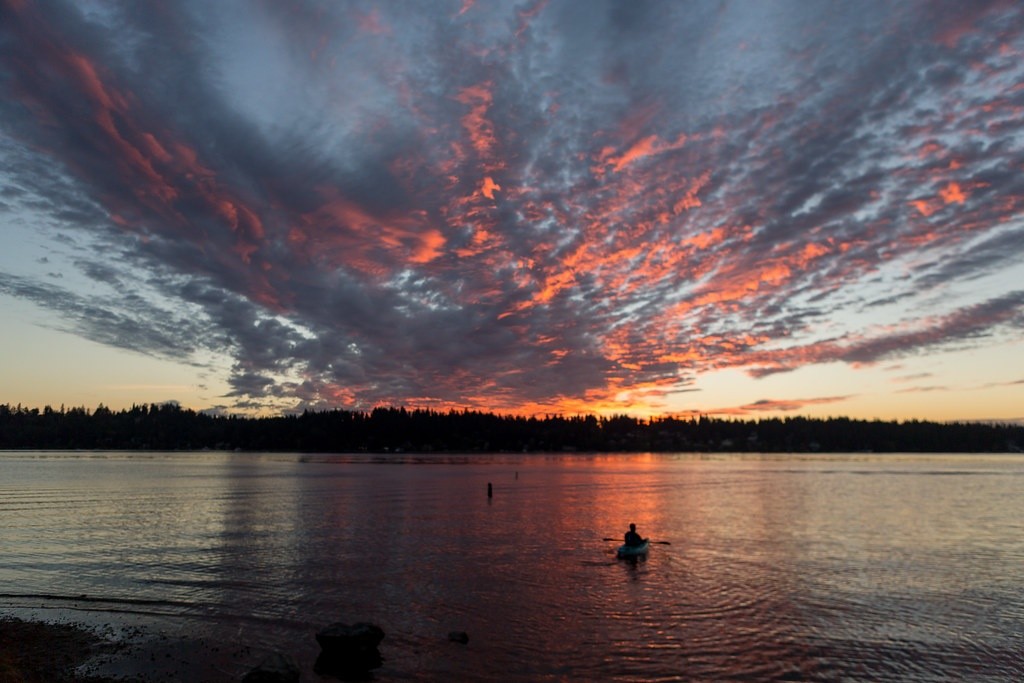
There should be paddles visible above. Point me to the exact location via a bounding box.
[603,537,672,547]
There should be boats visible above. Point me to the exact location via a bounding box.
[617,536,650,563]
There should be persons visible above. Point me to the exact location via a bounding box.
[625,523,648,548]
